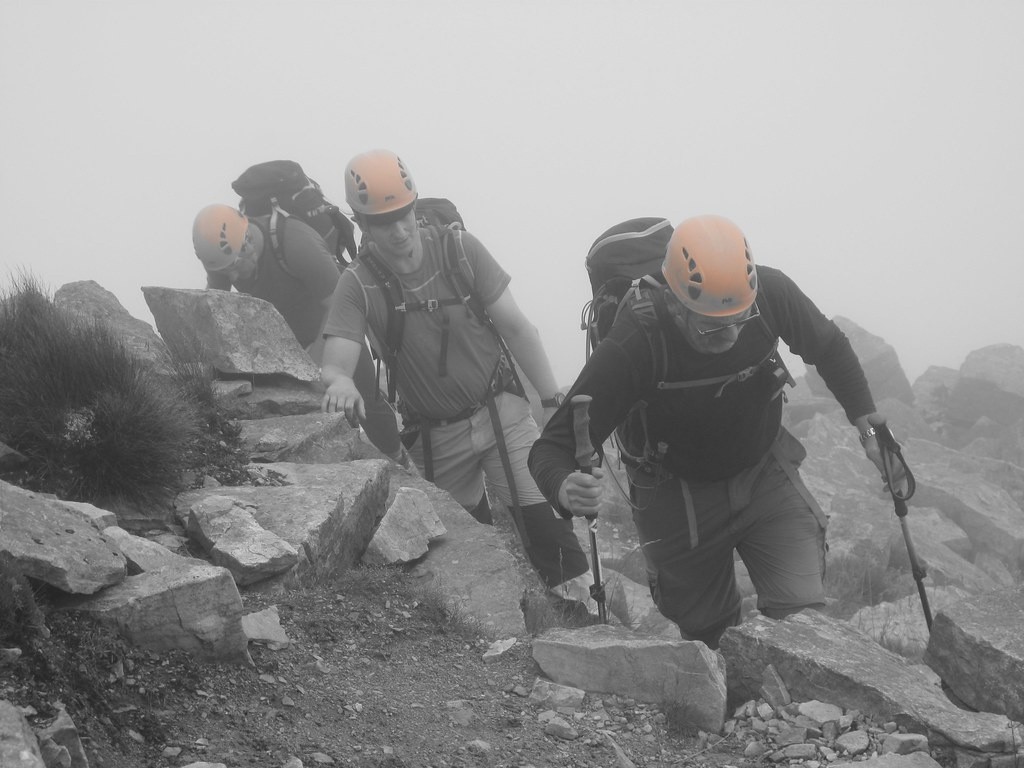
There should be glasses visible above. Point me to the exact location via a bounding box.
[672,301,760,341]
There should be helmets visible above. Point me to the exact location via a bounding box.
[192,204,249,269]
[345,150,417,214]
[663,215,758,316]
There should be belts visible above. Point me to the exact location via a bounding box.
[429,385,497,426]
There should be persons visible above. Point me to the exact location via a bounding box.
[324,147,595,620]
[524,218,900,653]
[192,205,411,479]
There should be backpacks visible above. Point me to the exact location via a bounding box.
[415,196,464,226]
[586,217,675,378]
[232,160,355,273]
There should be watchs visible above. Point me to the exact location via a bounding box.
[857,427,894,444]
[538,393,567,409]
